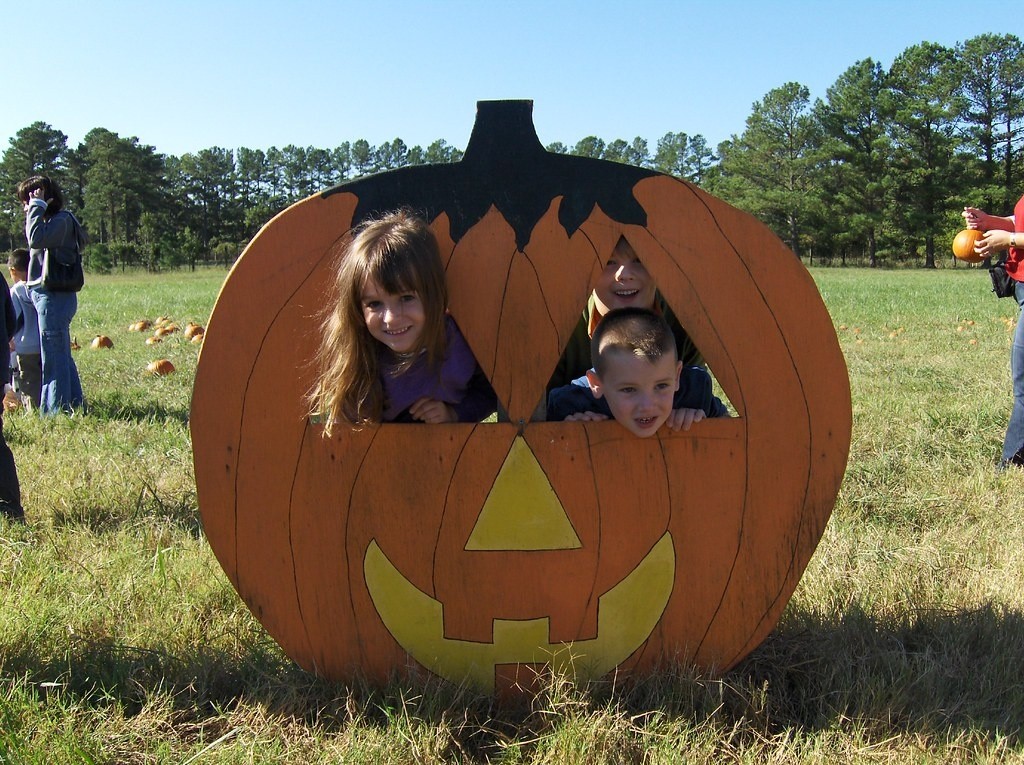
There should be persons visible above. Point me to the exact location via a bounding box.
[17,175,86,419]
[312,214,498,425]
[0,269,27,530]
[5,246,42,410]
[539,233,707,407]
[960,181,1024,469]
[545,304,731,438]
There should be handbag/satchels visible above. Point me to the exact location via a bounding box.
[43,215,84,292]
[988,260,1015,298]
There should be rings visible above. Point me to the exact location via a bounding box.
[986,251,992,256]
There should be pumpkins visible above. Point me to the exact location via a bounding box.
[128,317,204,344]
[148,360,173,375]
[92,335,113,348]
[952,230,987,263]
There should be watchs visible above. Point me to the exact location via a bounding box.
[1010,233,1016,247]
